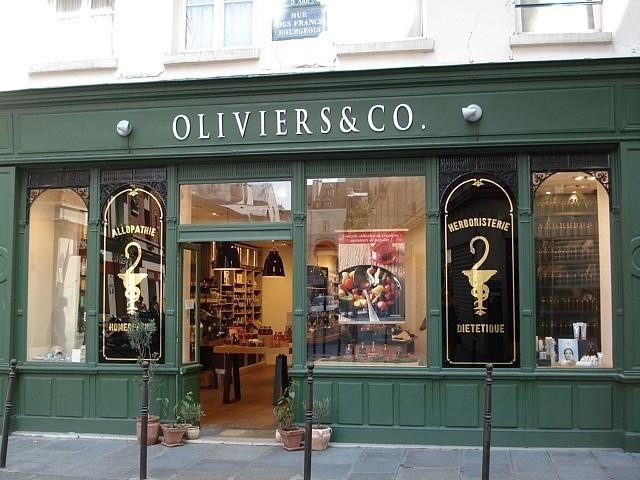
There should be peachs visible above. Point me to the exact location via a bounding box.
[345,279,354,291]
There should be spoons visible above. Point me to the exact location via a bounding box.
[353,266,381,322]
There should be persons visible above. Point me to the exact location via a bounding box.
[558,347,578,367]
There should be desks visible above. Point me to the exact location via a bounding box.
[212,344,291,407]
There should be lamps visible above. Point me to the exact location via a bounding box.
[261,241,286,277]
[212,241,241,271]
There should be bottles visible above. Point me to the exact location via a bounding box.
[396,347,402,358]
[258,325,273,349]
[345,344,353,355]
[274,331,289,348]
[359,341,366,353]
[370,340,378,353]
[383,344,389,354]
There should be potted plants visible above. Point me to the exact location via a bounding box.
[126,310,206,449]
[274,388,333,452]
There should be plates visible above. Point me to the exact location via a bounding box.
[340,265,404,322]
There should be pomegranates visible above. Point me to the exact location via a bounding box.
[377,301,386,312]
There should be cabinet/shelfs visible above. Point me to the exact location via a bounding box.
[199,240,264,348]
